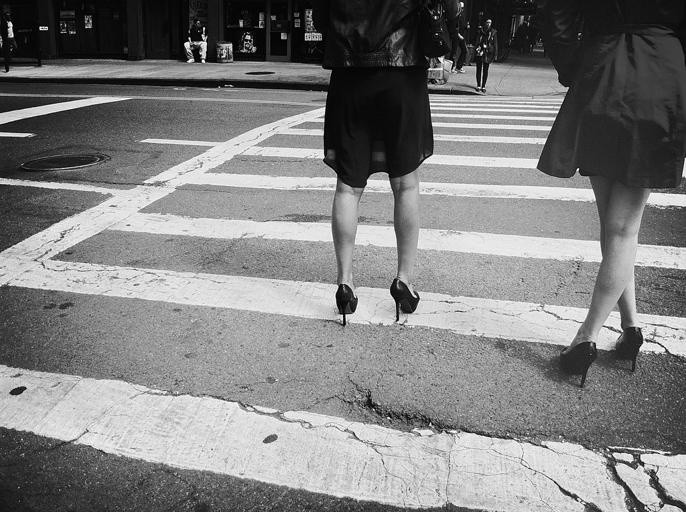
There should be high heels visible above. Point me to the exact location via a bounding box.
[615,327,643,372]
[336,283,358,326]
[390,278,419,321]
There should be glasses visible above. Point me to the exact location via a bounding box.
[245,39,252,43]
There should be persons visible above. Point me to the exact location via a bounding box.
[449,1,497,93]
[182,19,210,63]
[516,1,686,389]
[313,0,460,327]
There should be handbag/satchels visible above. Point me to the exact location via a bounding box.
[561,341,598,388]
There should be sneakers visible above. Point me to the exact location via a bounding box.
[187,58,194,64]
[201,59,205,63]
[476,87,486,93]
[449,68,466,73]
